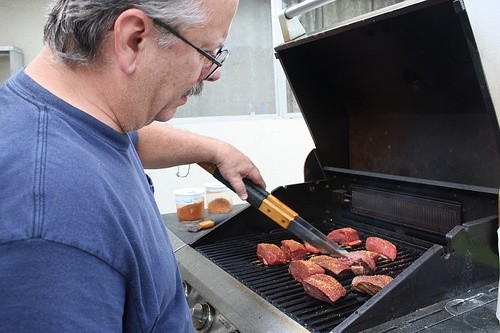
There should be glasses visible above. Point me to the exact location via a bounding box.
[147,14,230,81]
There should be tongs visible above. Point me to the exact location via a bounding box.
[197,162,350,259]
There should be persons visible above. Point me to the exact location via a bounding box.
[0,0,266,333]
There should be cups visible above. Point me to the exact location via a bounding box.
[175,188,206,224]
[204,181,233,214]
